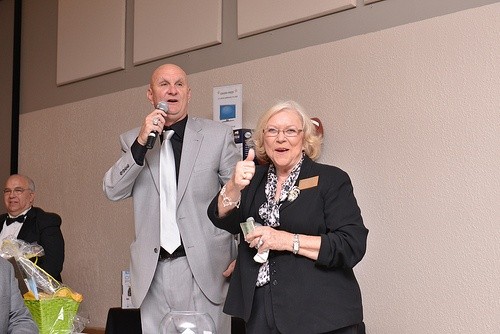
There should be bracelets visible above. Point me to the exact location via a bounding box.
[294,234,299,254]
[220,185,240,208]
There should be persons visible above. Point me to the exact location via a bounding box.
[0,258,39,334]
[103,64,241,334]
[207,101,370,334]
[0,176,64,296]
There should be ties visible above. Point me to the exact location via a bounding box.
[159,129,182,254]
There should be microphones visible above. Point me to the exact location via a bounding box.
[146,101,169,149]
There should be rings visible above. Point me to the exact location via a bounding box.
[255,240,263,249]
[153,118,158,124]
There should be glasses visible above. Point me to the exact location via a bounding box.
[3,188,31,195]
[264,126,302,137]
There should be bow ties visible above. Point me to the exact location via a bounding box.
[6,214,27,226]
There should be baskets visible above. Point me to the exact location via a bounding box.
[25,298,81,334]
